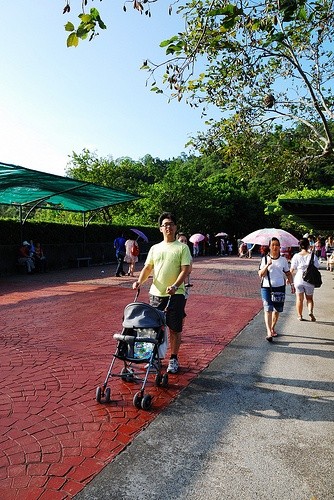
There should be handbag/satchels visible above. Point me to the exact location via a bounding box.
[131,240,140,256]
[271,292,285,302]
[302,252,322,288]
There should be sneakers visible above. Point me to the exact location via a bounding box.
[166,359,178,373]
[145,362,160,372]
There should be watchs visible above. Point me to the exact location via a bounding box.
[172,284,178,291]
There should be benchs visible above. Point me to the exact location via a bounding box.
[16,263,46,275]
[74,257,92,268]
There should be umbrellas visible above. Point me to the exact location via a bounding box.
[215,231,228,237]
[240,228,302,264]
[129,228,149,242]
[189,233,205,243]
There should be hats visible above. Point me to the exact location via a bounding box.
[22,240,30,246]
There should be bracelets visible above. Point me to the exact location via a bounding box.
[291,283,294,286]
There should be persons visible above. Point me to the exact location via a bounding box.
[257,237,297,342]
[132,211,192,374]
[289,239,321,322]
[18,240,55,273]
[175,232,190,290]
[123,234,139,277]
[172,233,334,287]
[113,233,126,277]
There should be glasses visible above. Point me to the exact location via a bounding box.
[160,221,175,228]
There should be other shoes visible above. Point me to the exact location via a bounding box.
[270,328,278,337]
[309,313,316,322]
[185,283,193,287]
[265,336,273,343]
[298,315,302,320]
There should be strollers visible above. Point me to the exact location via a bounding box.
[96,287,172,410]
